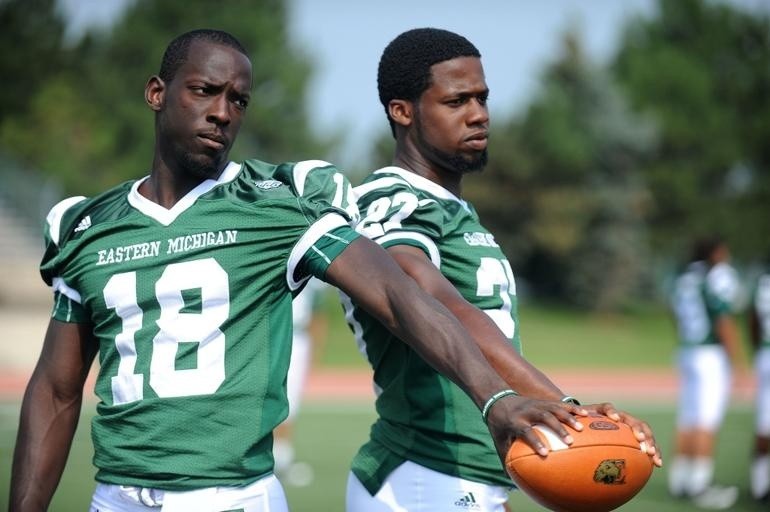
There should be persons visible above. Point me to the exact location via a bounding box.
[746,269,770,499]
[668,232,759,510]
[8,28,587,512]
[340,28,662,511]
[269,273,327,471]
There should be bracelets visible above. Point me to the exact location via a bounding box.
[560,396,582,406]
[482,390,519,426]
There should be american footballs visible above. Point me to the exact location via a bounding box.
[506,413,654,511]
